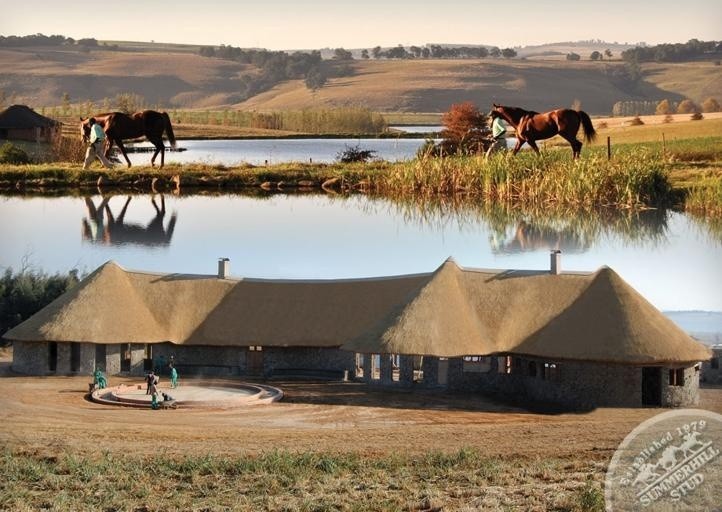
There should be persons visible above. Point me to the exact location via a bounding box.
[485,110,507,161]
[81,195,114,247]
[93,366,108,389]
[144,355,179,409]
[83,118,114,170]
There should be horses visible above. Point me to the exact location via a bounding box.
[79,192,179,248]
[78,108,177,172]
[487,215,596,257]
[486,101,598,160]
